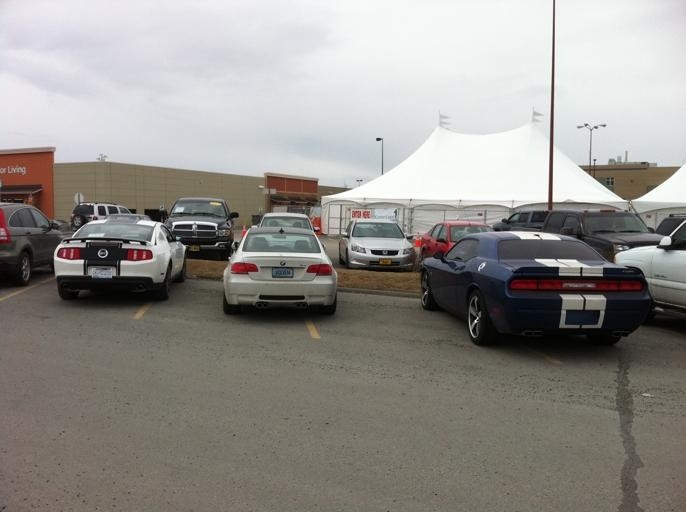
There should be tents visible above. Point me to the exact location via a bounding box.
[320,121,630,239]
[631,162,686,231]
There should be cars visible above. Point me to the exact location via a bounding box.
[418,209,686,347]
[338,217,416,272]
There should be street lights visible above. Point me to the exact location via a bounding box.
[357,180,362,187]
[578,123,607,174]
[376,137,384,175]
